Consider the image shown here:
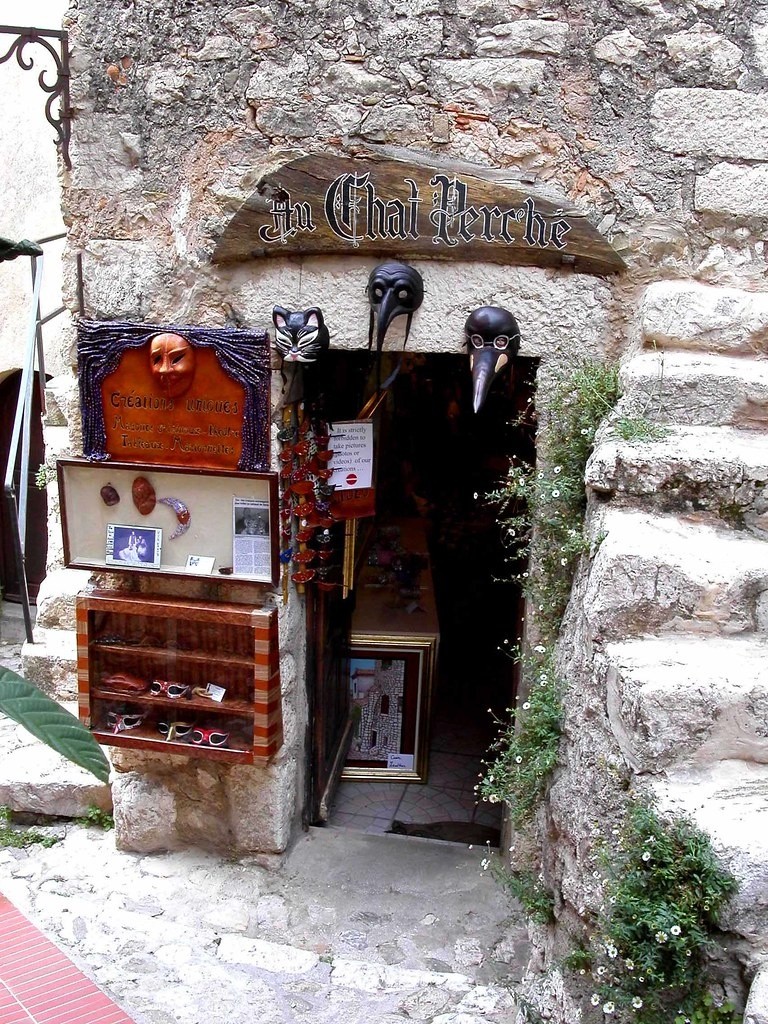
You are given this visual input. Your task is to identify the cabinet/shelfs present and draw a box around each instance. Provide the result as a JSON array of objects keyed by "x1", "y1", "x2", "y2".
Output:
[{"x1": 74, "y1": 595, "x2": 279, "y2": 766}]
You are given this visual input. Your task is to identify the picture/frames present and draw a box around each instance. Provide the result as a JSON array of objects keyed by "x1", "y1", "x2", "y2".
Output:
[{"x1": 341, "y1": 631, "x2": 437, "y2": 785}]
